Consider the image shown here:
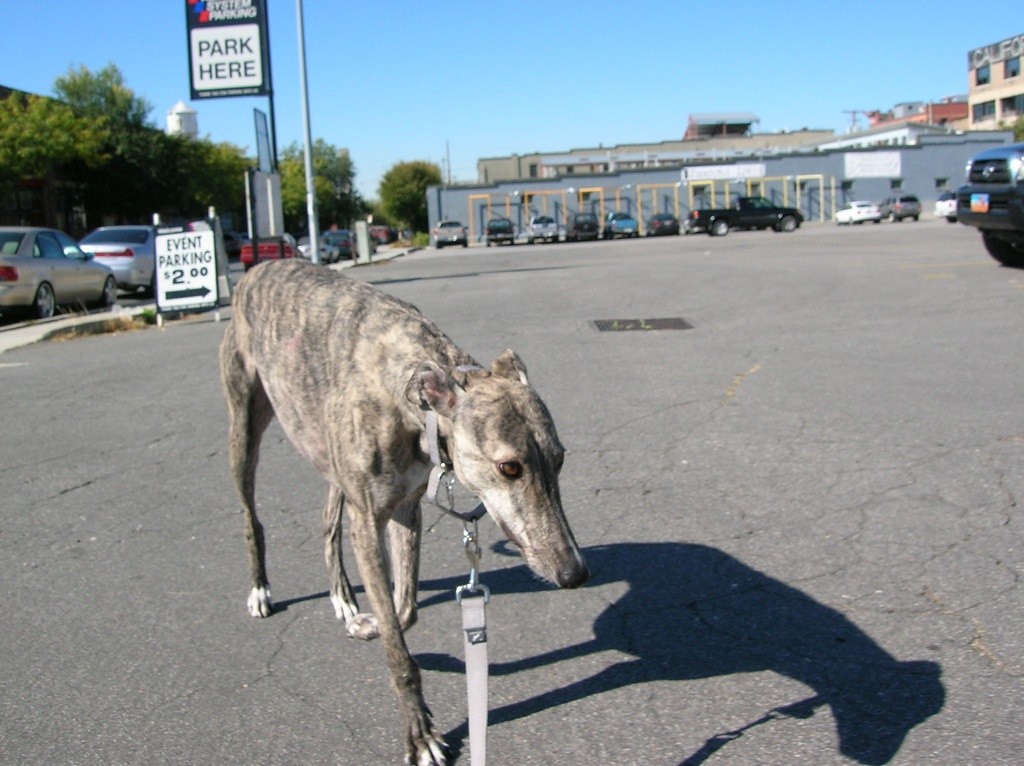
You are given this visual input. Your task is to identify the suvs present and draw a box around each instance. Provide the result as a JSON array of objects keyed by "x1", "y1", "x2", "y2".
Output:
[
  {"x1": 955, "y1": 141, "x2": 1024, "y2": 270},
  {"x1": 878, "y1": 195, "x2": 921, "y2": 223},
  {"x1": 433, "y1": 220, "x2": 468, "y2": 249}
]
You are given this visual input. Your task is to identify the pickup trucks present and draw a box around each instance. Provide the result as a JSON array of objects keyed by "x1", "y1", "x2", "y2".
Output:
[
  {"x1": 935, "y1": 198, "x2": 959, "y2": 223},
  {"x1": 689, "y1": 196, "x2": 804, "y2": 236},
  {"x1": 527, "y1": 216, "x2": 559, "y2": 244},
  {"x1": 602, "y1": 211, "x2": 639, "y2": 240}
]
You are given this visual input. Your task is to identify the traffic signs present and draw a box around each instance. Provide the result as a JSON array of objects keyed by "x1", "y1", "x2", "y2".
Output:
[{"x1": 155, "y1": 231, "x2": 217, "y2": 314}]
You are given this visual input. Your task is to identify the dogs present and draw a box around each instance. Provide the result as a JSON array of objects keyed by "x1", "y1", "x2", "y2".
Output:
[{"x1": 219, "y1": 256, "x2": 593, "y2": 766}]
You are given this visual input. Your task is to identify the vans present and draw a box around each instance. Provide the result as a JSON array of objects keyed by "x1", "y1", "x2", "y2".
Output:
[
  {"x1": 485, "y1": 219, "x2": 515, "y2": 246},
  {"x1": 565, "y1": 211, "x2": 599, "y2": 242}
]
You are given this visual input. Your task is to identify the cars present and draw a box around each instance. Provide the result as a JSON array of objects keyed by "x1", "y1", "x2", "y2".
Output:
[
  {"x1": 835, "y1": 200, "x2": 881, "y2": 226},
  {"x1": 644, "y1": 214, "x2": 679, "y2": 237},
  {"x1": 297, "y1": 225, "x2": 393, "y2": 265},
  {"x1": 241, "y1": 233, "x2": 302, "y2": 272},
  {"x1": 0, "y1": 225, "x2": 118, "y2": 322},
  {"x1": 62, "y1": 225, "x2": 166, "y2": 296}
]
